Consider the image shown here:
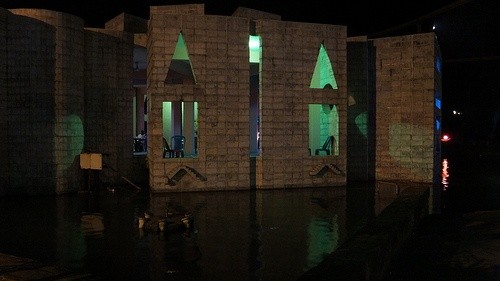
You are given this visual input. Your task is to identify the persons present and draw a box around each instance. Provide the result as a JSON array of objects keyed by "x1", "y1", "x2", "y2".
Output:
[{"x1": 137, "y1": 129, "x2": 146, "y2": 152}]
